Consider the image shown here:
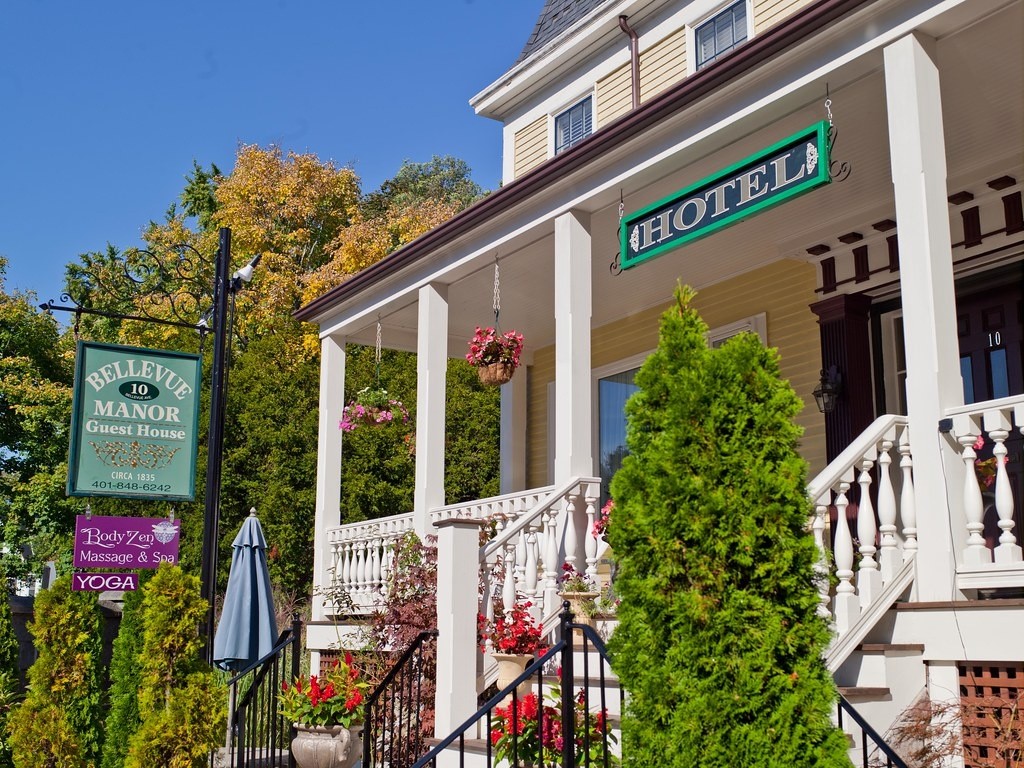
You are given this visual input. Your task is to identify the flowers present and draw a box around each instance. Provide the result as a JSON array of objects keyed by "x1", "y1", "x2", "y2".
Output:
[
  {"x1": 276, "y1": 650, "x2": 373, "y2": 729},
  {"x1": 465, "y1": 327, "x2": 525, "y2": 368},
  {"x1": 592, "y1": 499, "x2": 614, "y2": 540},
  {"x1": 478, "y1": 601, "x2": 549, "y2": 658},
  {"x1": 974, "y1": 436, "x2": 1009, "y2": 516},
  {"x1": 491, "y1": 666, "x2": 619, "y2": 768},
  {"x1": 339, "y1": 386, "x2": 409, "y2": 433},
  {"x1": 556, "y1": 563, "x2": 598, "y2": 618}
]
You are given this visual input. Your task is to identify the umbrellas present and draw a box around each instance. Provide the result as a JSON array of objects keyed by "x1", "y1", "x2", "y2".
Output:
[{"x1": 213, "y1": 506, "x2": 282, "y2": 680}]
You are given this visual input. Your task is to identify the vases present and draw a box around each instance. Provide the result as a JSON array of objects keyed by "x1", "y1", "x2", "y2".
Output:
[
  {"x1": 491, "y1": 653, "x2": 534, "y2": 708},
  {"x1": 559, "y1": 592, "x2": 600, "y2": 643},
  {"x1": 291, "y1": 723, "x2": 364, "y2": 768},
  {"x1": 602, "y1": 536, "x2": 619, "y2": 583},
  {"x1": 362, "y1": 416, "x2": 386, "y2": 427},
  {"x1": 985, "y1": 537, "x2": 994, "y2": 563},
  {"x1": 478, "y1": 363, "x2": 515, "y2": 384}
]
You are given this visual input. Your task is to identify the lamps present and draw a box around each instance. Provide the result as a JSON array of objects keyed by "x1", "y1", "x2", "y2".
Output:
[{"x1": 812, "y1": 365, "x2": 847, "y2": 412}]
